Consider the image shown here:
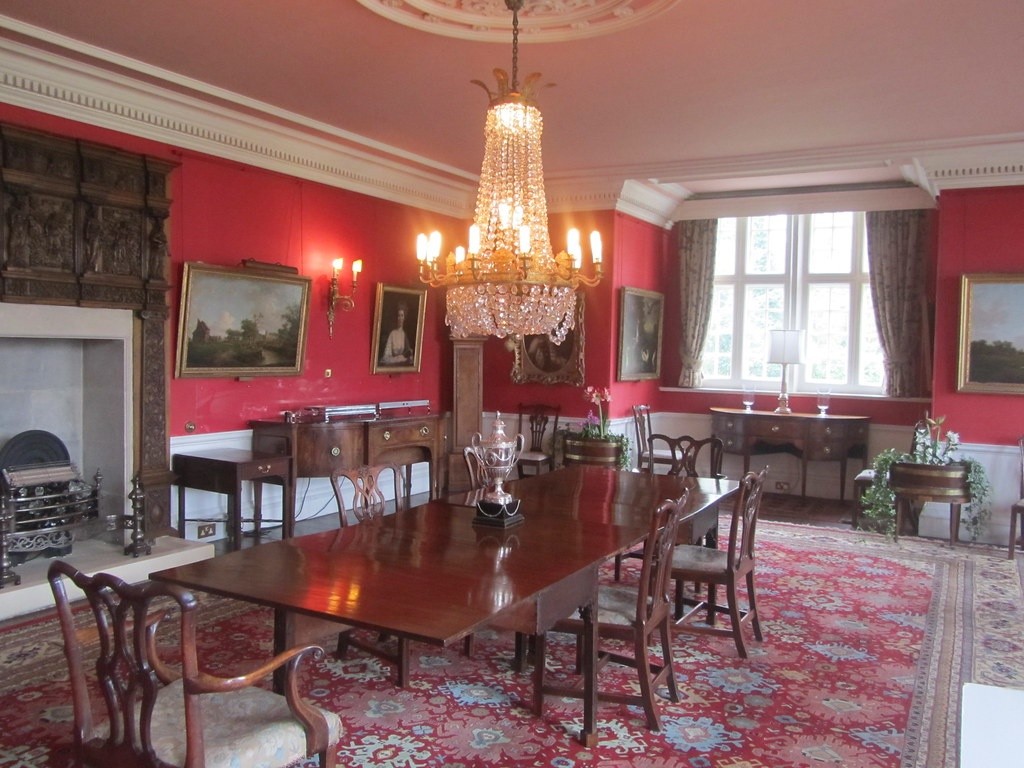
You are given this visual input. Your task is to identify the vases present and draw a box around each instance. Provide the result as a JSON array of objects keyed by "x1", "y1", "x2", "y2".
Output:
[{"x1": 562, "y1": 437, "x2": 622, "y2": 468}]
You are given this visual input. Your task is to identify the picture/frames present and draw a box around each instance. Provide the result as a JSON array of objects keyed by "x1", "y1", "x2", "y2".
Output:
[
  {"x1": 369, "y1": 282, "x2": 427, "y2": 373},
  {"x1": 510, "y1": 292, "x2": 585, "y2": 388},
  {"x1": 174, "y1": 262, "x2": 313, "y2": 377},
  {"x1": 955, "y1": 272, "x2": 1024, "y2": 396},
  {"x1": 617, "y1": 286, "x2": 665, "y2": 381}
]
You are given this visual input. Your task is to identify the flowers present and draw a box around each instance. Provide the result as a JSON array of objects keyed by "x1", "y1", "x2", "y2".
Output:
[{"x1": 549, "y1": 386, "x2": 631, "y2": 458}]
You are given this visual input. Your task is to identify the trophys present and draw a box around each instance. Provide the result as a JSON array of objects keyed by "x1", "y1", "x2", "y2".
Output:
[{"x1": 472, "y1": 411, "x2": 525, "y2": 527}]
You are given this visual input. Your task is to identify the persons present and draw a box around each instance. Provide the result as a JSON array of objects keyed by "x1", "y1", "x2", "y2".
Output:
[
  {"x1": 86, "y1": 212, "x2": 101, "y2": 263},
  {"x1": 10, "y1": 202, "x2": 67, "y2": 265},
  {"x1": 150, "y1": 223, "x2": 171, "y2": 280}
]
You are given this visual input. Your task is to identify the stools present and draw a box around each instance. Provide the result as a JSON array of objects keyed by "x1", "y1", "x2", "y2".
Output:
[
  {"x1": 1008, "y1": 499, "x2": 1024, "y2": 560},
  {"x1": 854, "y1": 468, "x2": 890, "y2": 528}
]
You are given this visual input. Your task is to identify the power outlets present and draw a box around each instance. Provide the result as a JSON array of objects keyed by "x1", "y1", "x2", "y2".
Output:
[
  {"x1": 198, "y1": 524, "x2": 216, "y2": 539},
  {"x1": 775, "y1": 482, "x2": 790, "y2": 490}
]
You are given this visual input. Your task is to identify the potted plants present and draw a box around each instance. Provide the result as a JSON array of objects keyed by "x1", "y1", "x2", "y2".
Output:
[{"x1": 859, "y1": 416, "x2": 993, "y2": 549}]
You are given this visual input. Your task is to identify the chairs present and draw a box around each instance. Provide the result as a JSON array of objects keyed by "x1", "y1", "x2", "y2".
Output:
[
  {"x1": 46, "y1": 563, "x2": 347, "y2": 768},
  {"x1": 331, "y1": 404, "x2": 770, "y2": 731}
]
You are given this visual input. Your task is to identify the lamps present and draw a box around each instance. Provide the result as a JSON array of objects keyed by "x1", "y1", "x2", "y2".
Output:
[
  {"x1": 415, "y1": 0, "x2": 605, "y2": 346},
  {"x1": 327, "y1": 257, "x2": 362, "y2": 339},
  {"x1": 766, "y1": 329, "x2": 801, "y2": 414}
]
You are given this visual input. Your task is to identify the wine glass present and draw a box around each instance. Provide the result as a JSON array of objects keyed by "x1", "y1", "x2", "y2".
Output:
[
  {"x1": 817, "y1": 390, "x2": 830, "y2": 417},
  {"x1": 742, "y1": 384, "x2": 756, "y2": 412}
]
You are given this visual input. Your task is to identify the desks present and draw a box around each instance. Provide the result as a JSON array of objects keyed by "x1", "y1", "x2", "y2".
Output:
[
  {"x1": 147, "y1": 463, "x2": 750, "y2": 748},
  {"x1": 248, "y1": 411, "x2": 448, "y2": 536},
  {"x1": 708, "y1": 407, "x2": 871, "y2": 505},
  {"x1": 172, "y1": 449, "x2": 295, "y2": 553}
]
